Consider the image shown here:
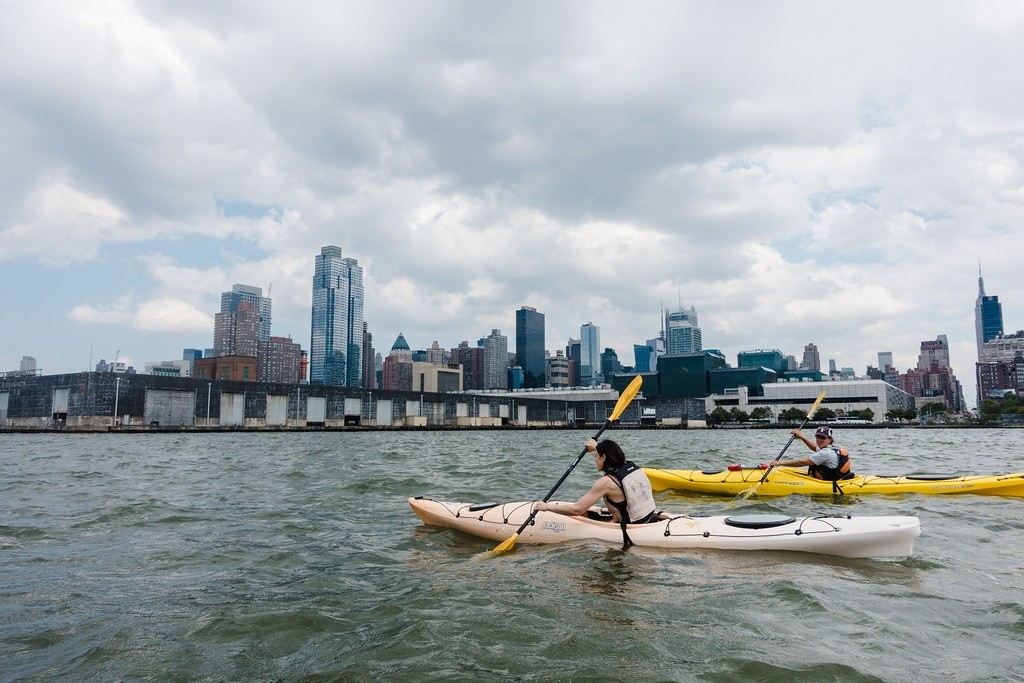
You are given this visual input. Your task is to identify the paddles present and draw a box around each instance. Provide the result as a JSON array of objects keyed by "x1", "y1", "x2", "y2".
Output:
[
  {"x1": 743, "y1": 391, "x2": 826, "y2": 499},
  {"x1": 492, "y1": 373, "x2": 643, "y2": 552}
]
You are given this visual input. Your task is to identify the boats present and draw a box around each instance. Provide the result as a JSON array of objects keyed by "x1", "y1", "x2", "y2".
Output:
[
  {"x1": 642, "y1": 466, "x2": 1024, "y2": 500},
  {"x1": 406, "y1": 496, "x2": 922, "y2": 560}
]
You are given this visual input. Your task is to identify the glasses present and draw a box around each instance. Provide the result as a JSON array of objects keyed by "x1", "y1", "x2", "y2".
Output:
[{"x1": 816, "y1": 435, "x2": 829, "y2": 440}]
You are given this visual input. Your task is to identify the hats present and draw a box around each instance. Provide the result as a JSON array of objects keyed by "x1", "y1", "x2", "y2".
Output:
[{"x1": 814, "y1": 426, "x2": 833, "y2": 439}]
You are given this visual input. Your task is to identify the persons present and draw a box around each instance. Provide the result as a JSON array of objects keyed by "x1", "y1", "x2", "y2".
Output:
[
  {"x1": 770, "y1": 427, "x2": 851, "y2": 481},
  {"x1": 533, "y1": 439, "x2": 658, "y2": 524}
]
